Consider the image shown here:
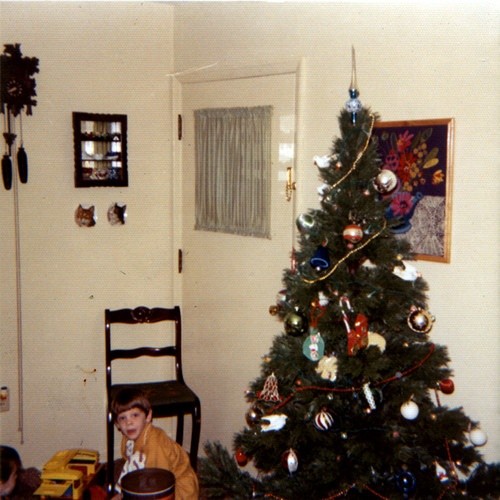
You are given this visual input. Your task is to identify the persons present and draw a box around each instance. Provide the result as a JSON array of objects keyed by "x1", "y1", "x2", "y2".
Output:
[
  {"x1": 0, "y1": 446, "x2": 20, "y2": 500},
  {"x1": 107, "y1": 389, "x2": 199, "y2": 500}
]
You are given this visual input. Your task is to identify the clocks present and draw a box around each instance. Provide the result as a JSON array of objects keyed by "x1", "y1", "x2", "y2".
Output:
[{"x1": 0, "y1": 43, "x2": 39, "y2": 190}]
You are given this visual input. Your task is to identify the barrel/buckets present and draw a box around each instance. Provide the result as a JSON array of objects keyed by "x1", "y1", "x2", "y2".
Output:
[{"x1": 120, "y1": 468, "x2": 176, "y2": 500}]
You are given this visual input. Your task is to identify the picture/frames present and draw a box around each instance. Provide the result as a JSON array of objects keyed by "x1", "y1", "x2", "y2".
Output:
[{"x1": 367, "y1": 118, "x2": 456, "y2": 264}]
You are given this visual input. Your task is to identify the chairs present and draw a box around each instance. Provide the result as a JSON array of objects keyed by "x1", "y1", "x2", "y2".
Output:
[{"x1": 104, "y1": 305, "x2": 202, "y2": 479}]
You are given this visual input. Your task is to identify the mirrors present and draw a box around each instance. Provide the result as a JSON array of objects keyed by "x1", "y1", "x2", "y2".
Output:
[{"x1": 71, "y1": 111, "x2": 129, "y2": 188}]
